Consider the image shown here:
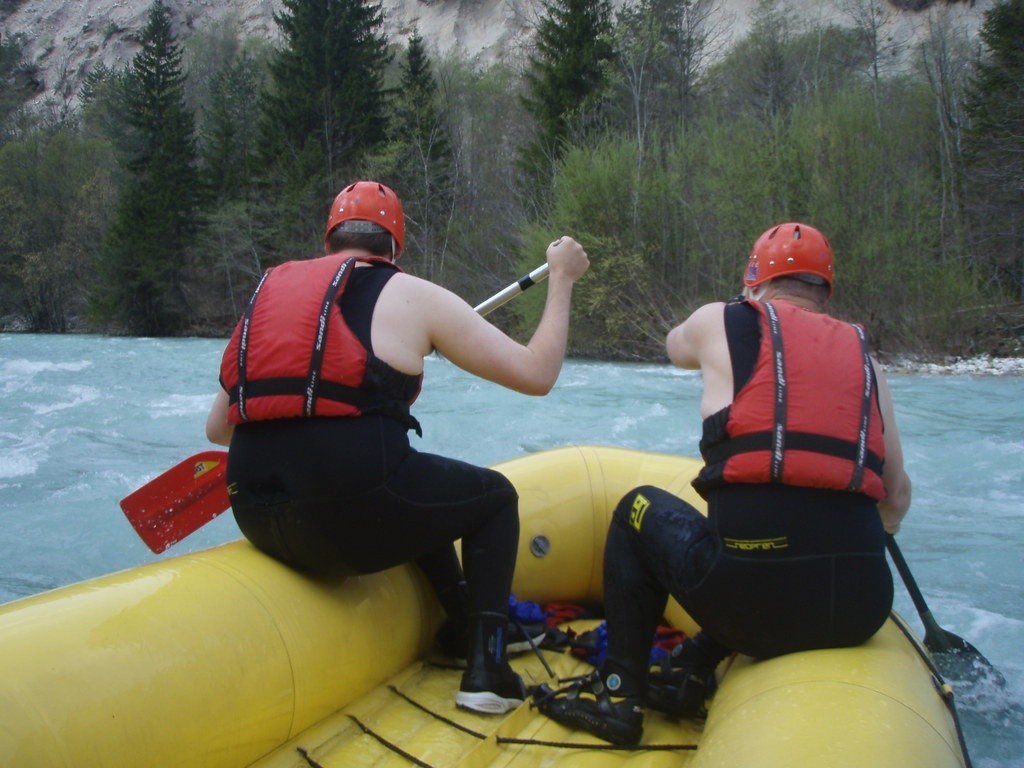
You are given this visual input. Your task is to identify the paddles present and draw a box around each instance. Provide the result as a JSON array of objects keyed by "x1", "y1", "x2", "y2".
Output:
[
  {"x1": 118, "y1": 260, "x2": 549, "y2": 554},
  {"x1": 884, "y1": 531, "x2": 1006, "y2": 691}
]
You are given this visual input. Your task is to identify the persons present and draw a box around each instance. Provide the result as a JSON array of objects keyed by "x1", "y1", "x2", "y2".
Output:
[
  {"x1": 206, "y1": 181, "x2": 589, "y2": 713},
  {"x1": 537, "y1": 223, "x2": 913, "y2": 751}
]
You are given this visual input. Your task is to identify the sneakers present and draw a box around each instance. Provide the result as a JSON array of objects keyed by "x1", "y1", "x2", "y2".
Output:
[
  {"x1": 447, "y1": 620, "x2": 546, "y2": 666},
  {"x1": 534, "y1": 683, "x2": 643, "y2": 746},
  {"x1": 641, "y1": 655, "x2": 706, "y2": 718},
  {"x1": 456, "y1": 665, "x2": 528, "y2": 713}
]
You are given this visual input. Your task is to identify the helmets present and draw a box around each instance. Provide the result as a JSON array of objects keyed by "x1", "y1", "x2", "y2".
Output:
[
  {"x1": 325, "y1": 181, "x2": 404, "y2": 251},
  {"x1": 744, "y1": 222, "x2": 834, "y2": 305}
]
